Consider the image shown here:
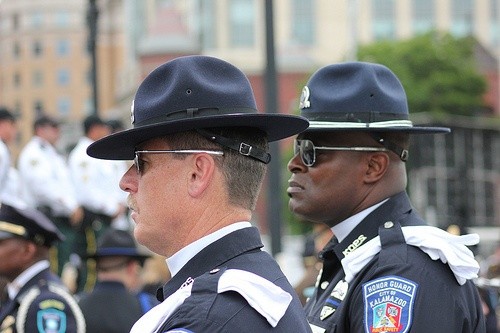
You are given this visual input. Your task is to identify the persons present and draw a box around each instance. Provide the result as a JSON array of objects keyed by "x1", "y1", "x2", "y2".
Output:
[
  {"x1": 40, "y1": 189, "x2": 176, "y2": 333},
  {"x1": 0, "y1": 200, "x2": 87, "y2": 333},
  {"x1": 291, "y1": 209, "x2": 500, "y2": 333},
  {"x1": 105, "y1": 118, "x2": 134, "y2": 219},
  {"x1": 67, "y1": 112, "x2": 125, "y2": 293},
  {"x1": 86, "y1": 54, "x2": 314, "y2": 333},
  {"x1": 284, "y1": 61, "x2": 487, "y2": 333},
  {"x1": 18, "y1": 115, "x2": 84, "y2": 285},
  {"x1": 0, "y1": 106, "x2": 28, "y2": 209}
]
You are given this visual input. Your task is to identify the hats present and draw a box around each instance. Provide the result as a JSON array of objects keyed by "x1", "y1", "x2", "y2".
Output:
[
  {"x1": 0, "y1": 201, "x2": 64, "y2": 246},
  {"x1": 81, "y1": 228, "x2": 155, "y2": 260},
  {"x1": 33, "y1": 116, "x2": 66, "y2": 125},
  {"x1": 301, "y1": 61, "x2": 451, "y2": 132},
  {"x1": 85, "y1": 55, "x2": 309, "y2": 161},
  {"x1": 83, "y1": 114, "x2": 112, "y2": 128},
  {"x1": 0, "y1": 108, "x2": 20, "y2": 121}
]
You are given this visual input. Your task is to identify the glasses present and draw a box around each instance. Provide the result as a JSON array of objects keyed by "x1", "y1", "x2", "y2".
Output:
[
  {"x1": 133, "y1": 145, "x2": 225, "y2": 171},
  {"x1": 293, "y1": 136, "x2": 390, "y2": 165}
]
